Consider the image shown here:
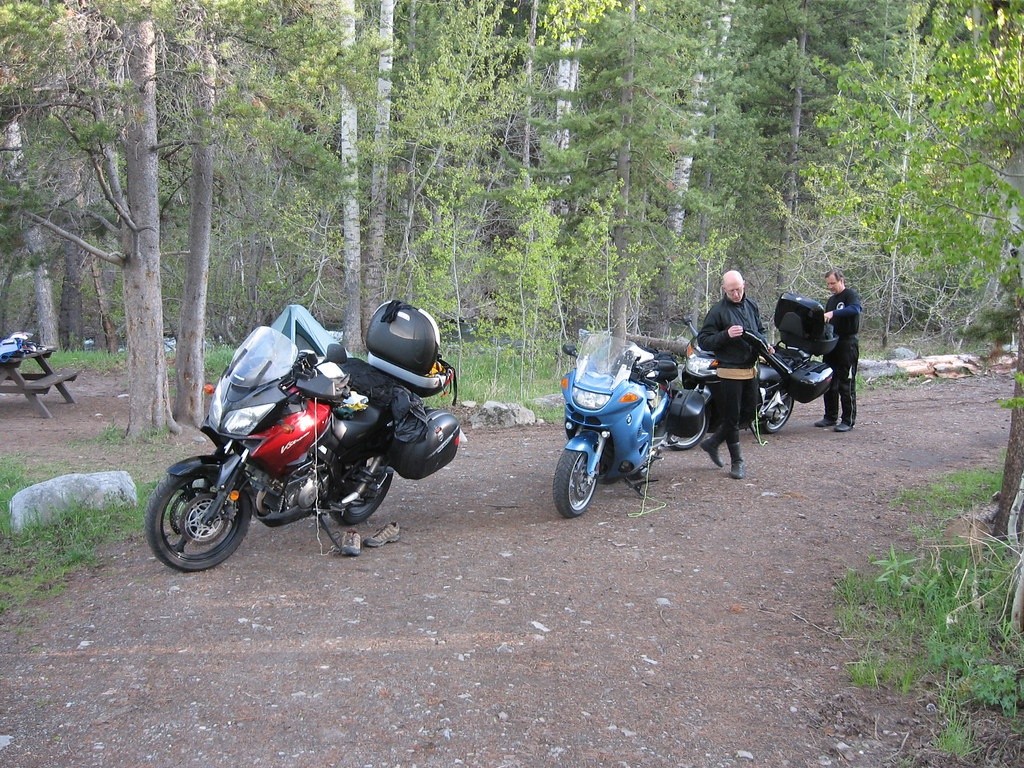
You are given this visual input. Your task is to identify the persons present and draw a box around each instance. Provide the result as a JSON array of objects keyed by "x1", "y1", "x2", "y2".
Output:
[
  {"x1": 696, "y1": 270, "x2": 775, "y2": 479},
  {"x1": 814, "y1": 268, "x2": 862, "y2": 432}
]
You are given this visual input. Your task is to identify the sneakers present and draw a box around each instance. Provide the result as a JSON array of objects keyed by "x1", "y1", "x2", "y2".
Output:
[
  {"x1": 814, "y1": 418, "x2": 838, "y2": 427},
  {"x1": 834, "y1": 422, "x2": 853, "y2": 432},
  {"x1": 363, "y1": 521, "x2": 401, "y2": 547},
  {"x1": 338, "y1": 528, "x2": 361, "y2": 556}
]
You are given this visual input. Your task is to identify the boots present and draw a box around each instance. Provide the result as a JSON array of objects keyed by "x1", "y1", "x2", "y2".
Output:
[
  {"x1": 725, "y1": 441, "x2": 745, "y2": 479},
  {"x1": 699, "y1": 425, "x2": 727, "y2": 468}
]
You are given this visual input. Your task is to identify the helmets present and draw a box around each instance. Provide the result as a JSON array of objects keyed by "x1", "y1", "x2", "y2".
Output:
[{"x1": 9, "y1": 332, "x2": 34, "y2": 354}]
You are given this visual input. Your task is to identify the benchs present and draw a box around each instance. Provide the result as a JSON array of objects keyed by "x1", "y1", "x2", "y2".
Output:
[{"x1": 23, "y1": 368, "x2": 82, "y2": 394}]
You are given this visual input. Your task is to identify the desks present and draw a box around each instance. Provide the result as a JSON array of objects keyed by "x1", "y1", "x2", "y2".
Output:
[{"x1": 0, "y1": 346, "x2": 77, "y2": 418}]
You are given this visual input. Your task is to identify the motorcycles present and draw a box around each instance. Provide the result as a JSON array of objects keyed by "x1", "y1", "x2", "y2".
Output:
[
  {"x1": 670, "y1": 296, "x2": 841, "y2": 436},
  {"x1": 0, "y1": 329, "x2": 46, "y2": 354},
  {"x1": 144, "y1": 296, "x2": 463, "y2": 574},
  {"x1": 550, "y1": 327, "x2": 708, "y2": 520}
]
